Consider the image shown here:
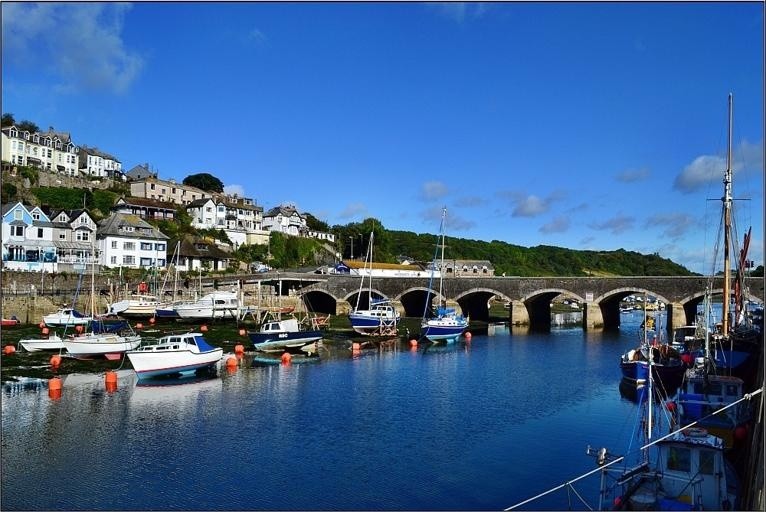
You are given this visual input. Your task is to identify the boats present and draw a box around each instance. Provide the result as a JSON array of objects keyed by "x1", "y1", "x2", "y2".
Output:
[
  {"x1": 125, "y1": 333, "x2": 224, "y2": 381},
  {"x1": 420, "y1": 206, "x2": 470, "y2": 345},
  {"x1": 19, "y1": 240, "x2": 142, "y2": 361},
  {"x1": 111, "y1": 239, "x2": 296, "y2": 321},
  {"x1": 348, "y1": 232, "x2": 401, "y2": 336},
  {"x1": 246, "y1": 283, "x2": 332, "y2": 354}
]
